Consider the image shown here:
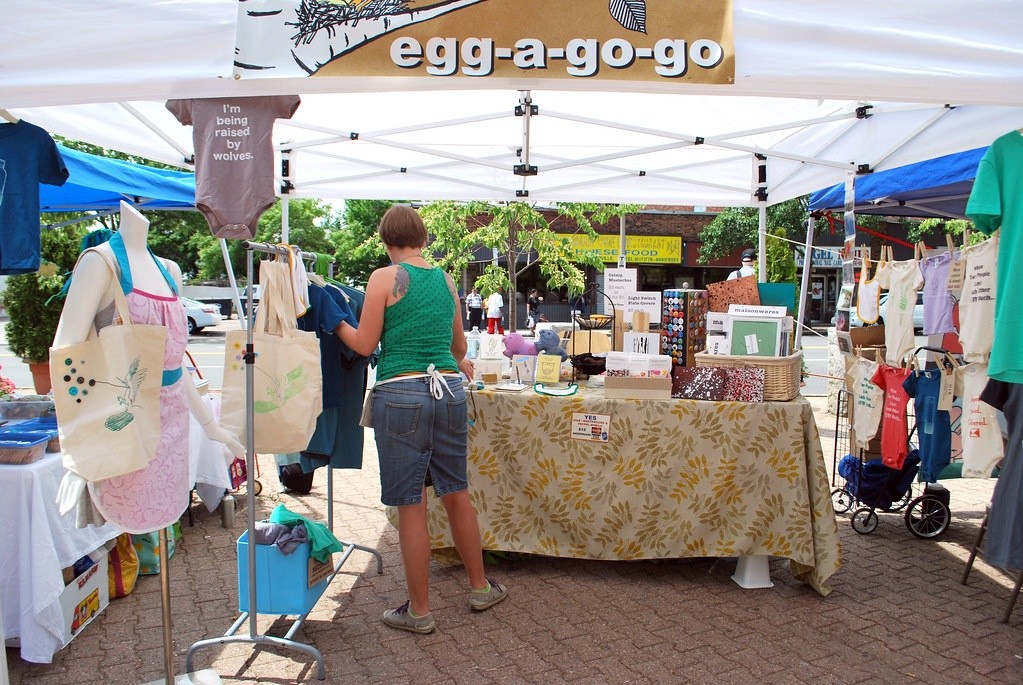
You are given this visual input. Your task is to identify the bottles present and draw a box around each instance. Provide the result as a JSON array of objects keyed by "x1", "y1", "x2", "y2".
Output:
[
  {"x1": 469, "y1": 327, "x2": 480, "y2": 339},
  {"x1": 221, "y1": 488, "x2": 235, "y2": 528}
]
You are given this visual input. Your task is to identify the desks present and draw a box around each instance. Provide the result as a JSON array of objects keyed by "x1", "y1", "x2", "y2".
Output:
[
  {"x1": 0, "y1": 392, "x2": 235, "y2": 663},
  {"x1": 385, "y1": 375, "x2": 841, "y2": 598}
]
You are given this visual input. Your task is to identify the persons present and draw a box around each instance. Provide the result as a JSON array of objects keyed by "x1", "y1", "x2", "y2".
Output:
[
  {"x1": 565, "y1": 290, "x2": 585, "y2": 314},
  {"x1": 465, "y1": 286, "x2": 482, "y2": 333},
  {"x1": 526, "y1": 289, "x2": 543, "y2": 335},
  {"x1": 481, "y1": 292, "x2": 504, "y2": 335},
  {"x1": 54, "y1": 199, "x2": 248, "y2": 530},
  {"x1": 726, "y1": 249, "x2": 757, "y2": 279},
  {"x1": 334, "y1": 205, "x2": 507, "y2": 634}
]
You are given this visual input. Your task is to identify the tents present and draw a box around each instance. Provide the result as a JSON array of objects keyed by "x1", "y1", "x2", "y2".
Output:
[
  {"x1": 37, "y1": 145, "x2": 248, "y2": 330},
  {"x1": 0, "y1": 0, "x2": 1023, "y2": 347},
  {"x1": 794, "y1": 146, "x2": 989, "y2": 350}
]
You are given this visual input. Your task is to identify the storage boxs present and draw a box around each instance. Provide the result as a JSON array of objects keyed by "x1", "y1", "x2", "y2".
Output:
[
  {"x1": 604, "y1": 375, "x2": 672, "y2": 400},
  {"x1": 59, "y1": 537, "x2": 118, "y2": 650},
  {"x1": 844, "y1": 325, "x2": 909, "y2": 463},
  {"x1": 237, "y1": 519, "x2": 327, "y2": 615}
]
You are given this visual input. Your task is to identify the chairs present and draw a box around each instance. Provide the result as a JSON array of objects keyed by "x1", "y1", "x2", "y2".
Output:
[{"x1": 960, "y1": 432, "x2": 1023, "y2": 624}]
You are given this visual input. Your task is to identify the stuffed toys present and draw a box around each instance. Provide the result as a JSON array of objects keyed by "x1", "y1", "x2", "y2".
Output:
[{"x1": 502, "y1": 329, "x2": 567, "y2": 362}]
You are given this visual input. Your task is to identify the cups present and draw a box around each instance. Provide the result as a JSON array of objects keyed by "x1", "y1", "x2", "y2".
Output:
[{"x1": 466, "y1": 339, "x2": 477, "y2": 359}]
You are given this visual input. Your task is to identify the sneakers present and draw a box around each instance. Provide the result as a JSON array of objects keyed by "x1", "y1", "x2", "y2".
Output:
[
  {"x1": 468, "y1": 577, "x2": 509, "y2": 611},
  {"x1": 381, "y1": 600, "x2": 436, "y2": 634}
]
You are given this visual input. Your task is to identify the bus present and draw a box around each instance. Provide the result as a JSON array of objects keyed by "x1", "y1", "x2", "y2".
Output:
[{"x1": 182, "y1": 296, "x2": 223, "y2": 335}]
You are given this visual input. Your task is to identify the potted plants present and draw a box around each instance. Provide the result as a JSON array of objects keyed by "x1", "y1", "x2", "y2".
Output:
[{"x1": 0, "y1": 216, "x2": 90, "y2": 397}]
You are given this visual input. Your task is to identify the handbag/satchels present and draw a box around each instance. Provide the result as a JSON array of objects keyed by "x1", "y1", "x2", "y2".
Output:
[
  {"x1": 49, "y1": 247, "x2": 167, "y2": 481},
  {"x1": 219, "y1": 263, "x2": 323, "y2": 453},
  {"x1": 525, "y1": 316, "x2": 535, "y2": 328},
  {"x1": 360, "y1": 384, "x2": 379, "y2": 428}
]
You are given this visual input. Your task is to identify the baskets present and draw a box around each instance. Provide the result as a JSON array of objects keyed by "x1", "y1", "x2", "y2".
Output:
[
  {"x1": 696, "y1": 350, "x2": 803, "y2": 401},
  {"x1": 0, "y1": 428, "x2": 61, "y2": 465}
]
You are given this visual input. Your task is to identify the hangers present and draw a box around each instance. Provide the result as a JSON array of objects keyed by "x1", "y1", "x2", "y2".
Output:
[{"x1": 259, "y1": 242, "x2": 346, "y2": 288}]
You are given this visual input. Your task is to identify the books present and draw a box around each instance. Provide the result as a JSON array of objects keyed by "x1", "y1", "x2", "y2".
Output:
[{"x1": 706, "y1": 312, "x2": 790, "y2": 357}]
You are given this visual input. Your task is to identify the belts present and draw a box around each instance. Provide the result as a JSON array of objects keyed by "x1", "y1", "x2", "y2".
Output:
[{"x1": 393, "y1": 369, "x2": 454, "y2": 380}]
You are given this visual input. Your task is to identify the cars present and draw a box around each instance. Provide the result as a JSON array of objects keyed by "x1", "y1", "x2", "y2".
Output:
[
  {"x1": 241, "y1": 284, "x2": 261, "y2": 314},
  {"x1": 831, "y1": 291, "x2": 925, "y2": 328}
]
